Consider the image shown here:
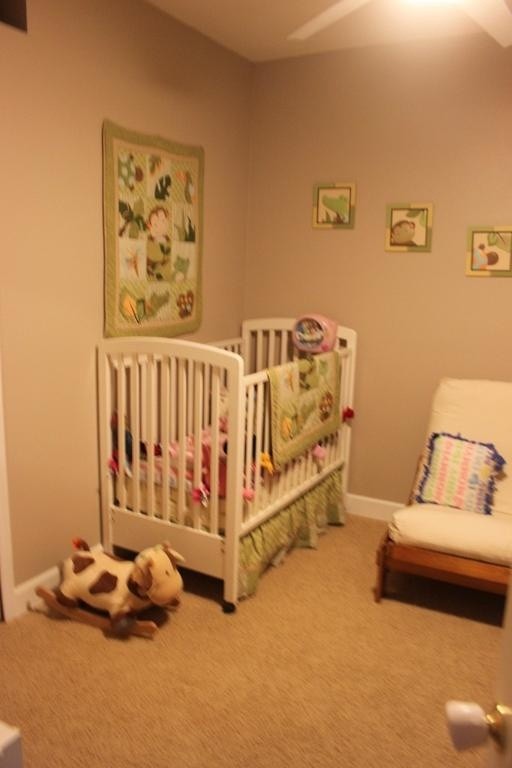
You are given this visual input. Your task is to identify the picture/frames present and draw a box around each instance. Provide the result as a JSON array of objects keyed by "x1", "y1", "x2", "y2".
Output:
[
  {"x1": 465, "y1": 224, "x2": 512, "y2": 278},
  {"x1": 312, "y1": 182, "x2": 356, "y2": 230},
  {"x1": 384, "y1": 203, "x2": 434, "y2": 252}
]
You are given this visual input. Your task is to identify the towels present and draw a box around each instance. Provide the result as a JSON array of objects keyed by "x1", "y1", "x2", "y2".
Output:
[{"x1": 267, "y1": 350, "x2": 344, "y2": 472}]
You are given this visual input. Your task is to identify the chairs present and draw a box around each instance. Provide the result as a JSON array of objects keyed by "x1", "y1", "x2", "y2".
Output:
[{"x1": 370, "y1": 378, "x2": 512, "y2": 627}]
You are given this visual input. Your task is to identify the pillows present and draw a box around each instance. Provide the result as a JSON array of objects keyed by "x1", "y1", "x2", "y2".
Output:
[{"x1": 416, "y1": 431, "x2": 506, "y2": 515}]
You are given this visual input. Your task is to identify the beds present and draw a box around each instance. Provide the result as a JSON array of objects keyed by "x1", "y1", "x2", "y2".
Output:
[{"x1": 96, "y1": 316, "x2": 357, "y2": 614}]
